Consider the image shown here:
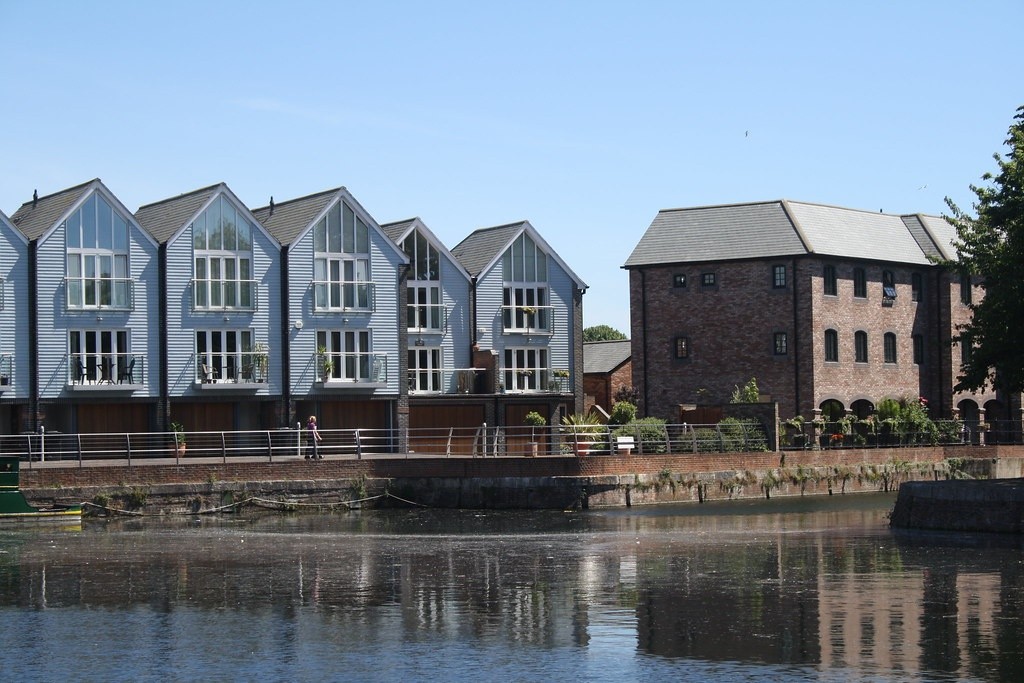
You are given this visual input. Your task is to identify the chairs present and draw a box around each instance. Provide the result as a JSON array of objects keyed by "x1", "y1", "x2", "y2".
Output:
[
  {"x1": 202, "y1": 364, "x2": 219, "y2": 384},
  {"x1": 237, "y1": 363, "x2": 256, "y2": 383},
  {"x1": 76, "y1": 360, "x2": 96, "y2": 385},
  {"x1": 120, "y1": 360, "x2": 135, "y2": 384}
]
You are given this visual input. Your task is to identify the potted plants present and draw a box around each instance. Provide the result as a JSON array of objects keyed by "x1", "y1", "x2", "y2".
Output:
[
  {"x1": 169, "y1": 421, "x2": 186, "y2": 458},
  {"x1": 523, "y1": 411, "x2": 546, "y2": 456},
  {"x1": 612, "y1": 401, "x2": 638, "y2": 455},
  {"x1": 563, "y1": 411, "x2": 602, "y2": 456},
  {"x1": 321, "y1": 360, "x2": 335, "y2": 382},
  {"x1": 252, "y1": 341, "x2": 271, "y2": 383}
]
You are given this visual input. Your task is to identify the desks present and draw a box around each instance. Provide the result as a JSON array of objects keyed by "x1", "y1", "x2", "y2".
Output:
[
  {"x1": 97, "y1": 364, "x2": 116, "y2": 385},
  {"x1": 222, "y1": 366, "x2": 240, "y2": 383}
]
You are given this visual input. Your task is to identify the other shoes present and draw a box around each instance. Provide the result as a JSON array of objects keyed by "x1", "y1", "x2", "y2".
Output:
[
  {"x1": 319, "y1": 455, "x2": 323, "y2": 459},
  {"x1": 305, "y1": 456, "x2": 310, "y2": 459}
]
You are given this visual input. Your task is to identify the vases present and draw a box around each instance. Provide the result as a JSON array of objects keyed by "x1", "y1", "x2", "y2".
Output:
[{"x1": 834, "y1": 442, "x2": 844, "y2": 447}]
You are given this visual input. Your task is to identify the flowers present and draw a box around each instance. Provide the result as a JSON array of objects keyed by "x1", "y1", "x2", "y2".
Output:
[{"x1": 831, "y1": 435, "x2": 844, "y2": 442}]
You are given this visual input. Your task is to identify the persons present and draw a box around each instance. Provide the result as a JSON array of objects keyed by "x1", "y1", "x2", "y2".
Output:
[{"x1": 304, "y1": 416, "x2": 325, "y2": 459}]
